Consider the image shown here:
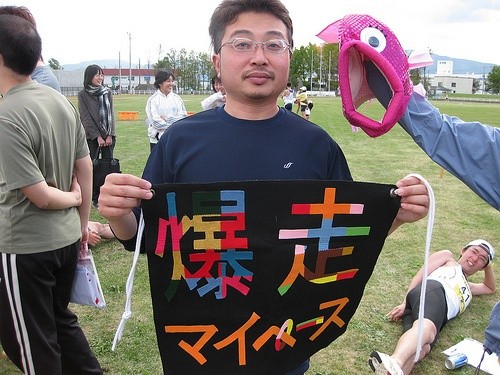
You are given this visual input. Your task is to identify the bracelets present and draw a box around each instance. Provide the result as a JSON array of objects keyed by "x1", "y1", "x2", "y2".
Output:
[{"x1": 484, "y1": 262, "x2": 495, "y2": 272}]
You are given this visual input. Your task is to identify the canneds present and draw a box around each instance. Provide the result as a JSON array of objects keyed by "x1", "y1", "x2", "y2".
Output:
[{"x1": 444, "y1": 353, "x2": 468, "y2": 370}]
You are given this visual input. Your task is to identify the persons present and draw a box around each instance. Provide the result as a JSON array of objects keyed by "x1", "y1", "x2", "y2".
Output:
[
  {"x1": 144, "y1": 71, "x2": 188, "y2": 153},
  {"x1": 98, "y1": 0, "x2": 429, "y2": 375},
  {"x1": 368, "y1": 238, "x2": 497, "y2": 375},
  {"x1": 0, "y1": 5, "x2": 63, "y2": 93},
  {"x1": 77, "y1": 64, "x2": 117, "y2": 208},
  {"x1": 358, "y1": 47, "x2": 500, "y2": 375},
  {"x1": 0, "y1": 14, "x2": 104, "y2": 375},
  {"x1": 211, "y1": 75, "x2": 227, "y2": 109},
  {"x1": 281, "y1": 83, "x2": 313, "y2": 120}
]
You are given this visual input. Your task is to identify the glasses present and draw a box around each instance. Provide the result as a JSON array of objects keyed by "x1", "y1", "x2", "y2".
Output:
[
  {"x1": 217, "y1": 37, "x2": 293, "y2": 54},
  {"x1": 92, "y1": 74, "x2": 104, "y2": 78}
]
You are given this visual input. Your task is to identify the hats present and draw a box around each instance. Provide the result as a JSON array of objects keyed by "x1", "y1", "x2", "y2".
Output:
[{"x1": 458, "y1": 240, "x2": 496, "y2": 272}]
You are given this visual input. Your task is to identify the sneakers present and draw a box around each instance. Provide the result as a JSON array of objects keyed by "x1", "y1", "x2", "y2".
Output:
[{"x1": 367, "y1": 350, "x2": 405, "y2": 375}]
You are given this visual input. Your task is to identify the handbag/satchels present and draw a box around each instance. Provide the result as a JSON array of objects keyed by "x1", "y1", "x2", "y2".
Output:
[{"x1": 91, "y1": 143, "x2": 122, "y2": 188}]
